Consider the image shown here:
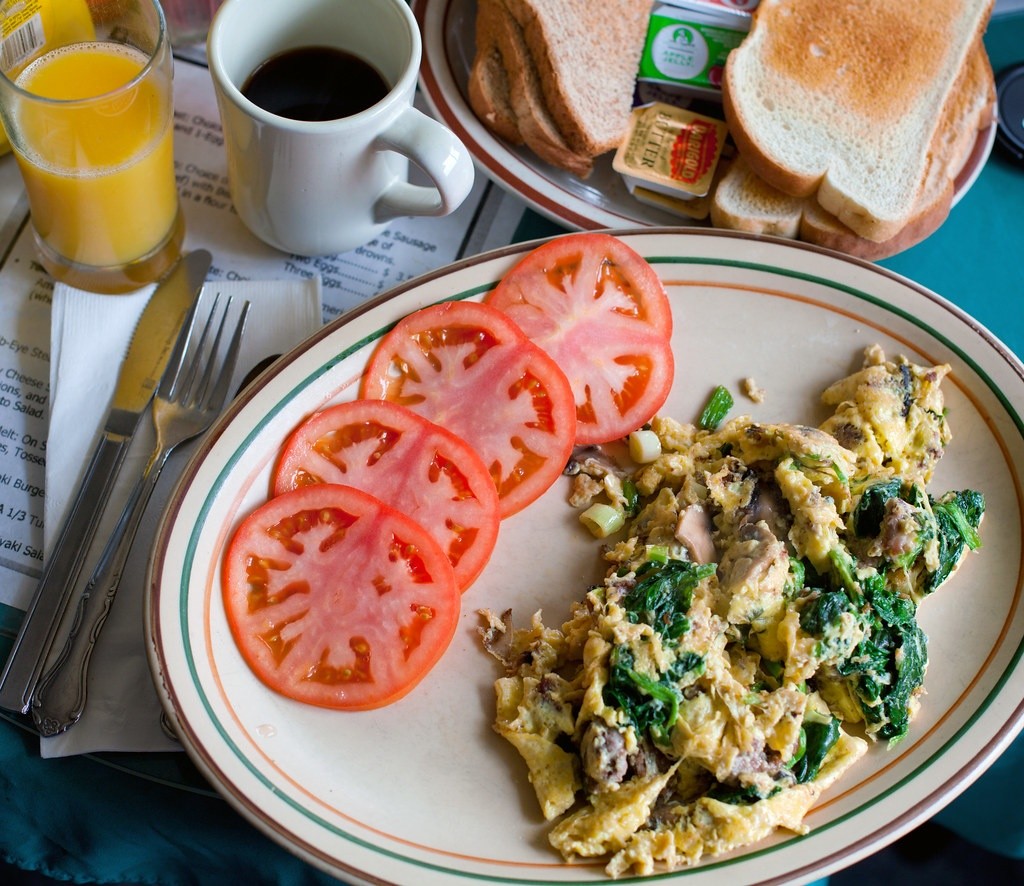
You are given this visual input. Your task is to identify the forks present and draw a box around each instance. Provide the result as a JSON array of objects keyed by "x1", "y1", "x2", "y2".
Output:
[{"x1": 30, "y1": 286, "x2": 251, "y2": 743}]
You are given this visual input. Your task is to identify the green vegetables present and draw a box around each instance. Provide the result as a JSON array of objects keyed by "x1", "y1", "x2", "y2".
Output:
[{"x1": 605, "y1": 476, "x2": 987, "y2": 805}]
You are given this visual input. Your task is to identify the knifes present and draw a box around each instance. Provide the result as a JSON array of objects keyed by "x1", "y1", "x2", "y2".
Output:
[{"x1": 1, "y1": 250, "x2": 211, "y2": 713}]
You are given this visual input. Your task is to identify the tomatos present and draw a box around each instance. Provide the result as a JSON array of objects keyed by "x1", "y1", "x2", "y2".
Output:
[{"x1": 220, "y1": 233, "x2": 675, "y2": 709}]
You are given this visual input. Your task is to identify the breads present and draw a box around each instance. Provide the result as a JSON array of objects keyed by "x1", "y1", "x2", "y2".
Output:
[{"x1": 466, "y1": 0, "x2": 998, "y2": 262}]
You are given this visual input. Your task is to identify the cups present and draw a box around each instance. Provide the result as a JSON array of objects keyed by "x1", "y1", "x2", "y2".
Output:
[
  {"x1": 207, "y1": 0, "x2": 475, "y2": 258},
  {"x1": 1, "y1": 0, "x2": 186, "y2": 295}
]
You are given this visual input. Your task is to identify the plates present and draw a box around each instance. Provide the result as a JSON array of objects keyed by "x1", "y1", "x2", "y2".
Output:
[
  {"x1": 409, "y1": 0, "x2": 1000, "y2": 260},
  {"x1": 146, "y1": 227, "x2": 1023, "y2": 886}
]
